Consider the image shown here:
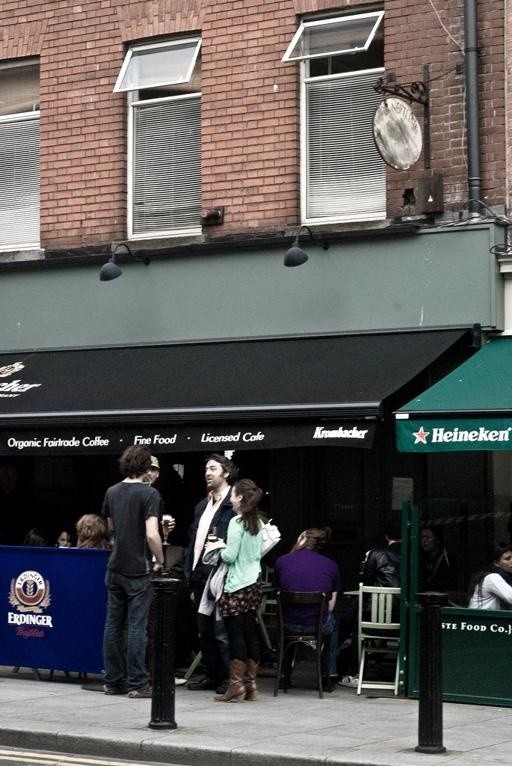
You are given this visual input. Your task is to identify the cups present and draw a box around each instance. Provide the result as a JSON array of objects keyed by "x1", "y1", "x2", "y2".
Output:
[{"x1": 207, "y1": 533, "x2": 218, "y2": 542}]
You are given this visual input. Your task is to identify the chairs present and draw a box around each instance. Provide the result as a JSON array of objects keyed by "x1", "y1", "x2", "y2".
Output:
[{"x1": 168, "y1": 566, "x2": 408, "y2": 699}]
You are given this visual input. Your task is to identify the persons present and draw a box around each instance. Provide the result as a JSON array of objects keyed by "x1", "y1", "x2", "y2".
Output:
[
  {"x1": 419, "y1": 528, "x2": 469, "y2": 593},
  {"x1": 466, "y1": 542, "x2": 512, "y2": 610},
  {"x1": 56, "y1": 446, "x2": 403, "y2": 702},
  {"x1": 24, "y1": 527, "x2": 51, "y2": 547}
]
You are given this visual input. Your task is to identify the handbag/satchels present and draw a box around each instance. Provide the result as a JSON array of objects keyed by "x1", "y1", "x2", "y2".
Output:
[{"x1": 260, "y1": 522, "x2": 282, "y2": 557}]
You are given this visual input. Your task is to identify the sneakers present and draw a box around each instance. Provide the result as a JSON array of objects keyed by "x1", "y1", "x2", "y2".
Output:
[
  {"x1": 104, "y1": 685, "x2": 131, "y2": 694},
  {"x1": 338, "y1": 676, "x2": 358, "y2": 688},
  {"x1": 129, "y1": 687, "x2": 153, "y2": 698},
  {"x1": 322, "y1": 677, "x2": 334, "y2": 691},
  {"x1": 279, "y1": 677, "x2": 291, "y2": 689}
]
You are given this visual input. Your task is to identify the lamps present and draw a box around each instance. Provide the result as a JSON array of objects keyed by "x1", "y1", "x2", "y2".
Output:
[
  {"x1": 281, "y1": 224, "x2": 330, "y2": 266},
  {"x1": 96, "y1": 243, "x2": 152, "y2": 282}
]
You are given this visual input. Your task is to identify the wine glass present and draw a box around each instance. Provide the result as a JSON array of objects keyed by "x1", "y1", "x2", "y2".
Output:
[{"x1": 161, "y1": 516, "x2": 172, "y2": 546}]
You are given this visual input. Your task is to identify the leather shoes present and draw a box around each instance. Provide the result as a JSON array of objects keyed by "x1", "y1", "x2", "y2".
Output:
[
  {"x1": 175, "y1": 676, "x2": 187, "y2": 685},
  {"x1": 188, "y1": 675, "x2": 217, "y2": 689}
]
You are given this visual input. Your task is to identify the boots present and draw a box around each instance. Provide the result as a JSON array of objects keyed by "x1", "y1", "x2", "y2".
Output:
[{"x1": 215, "y1": 658, "x2": 260, "y2": 701}]
